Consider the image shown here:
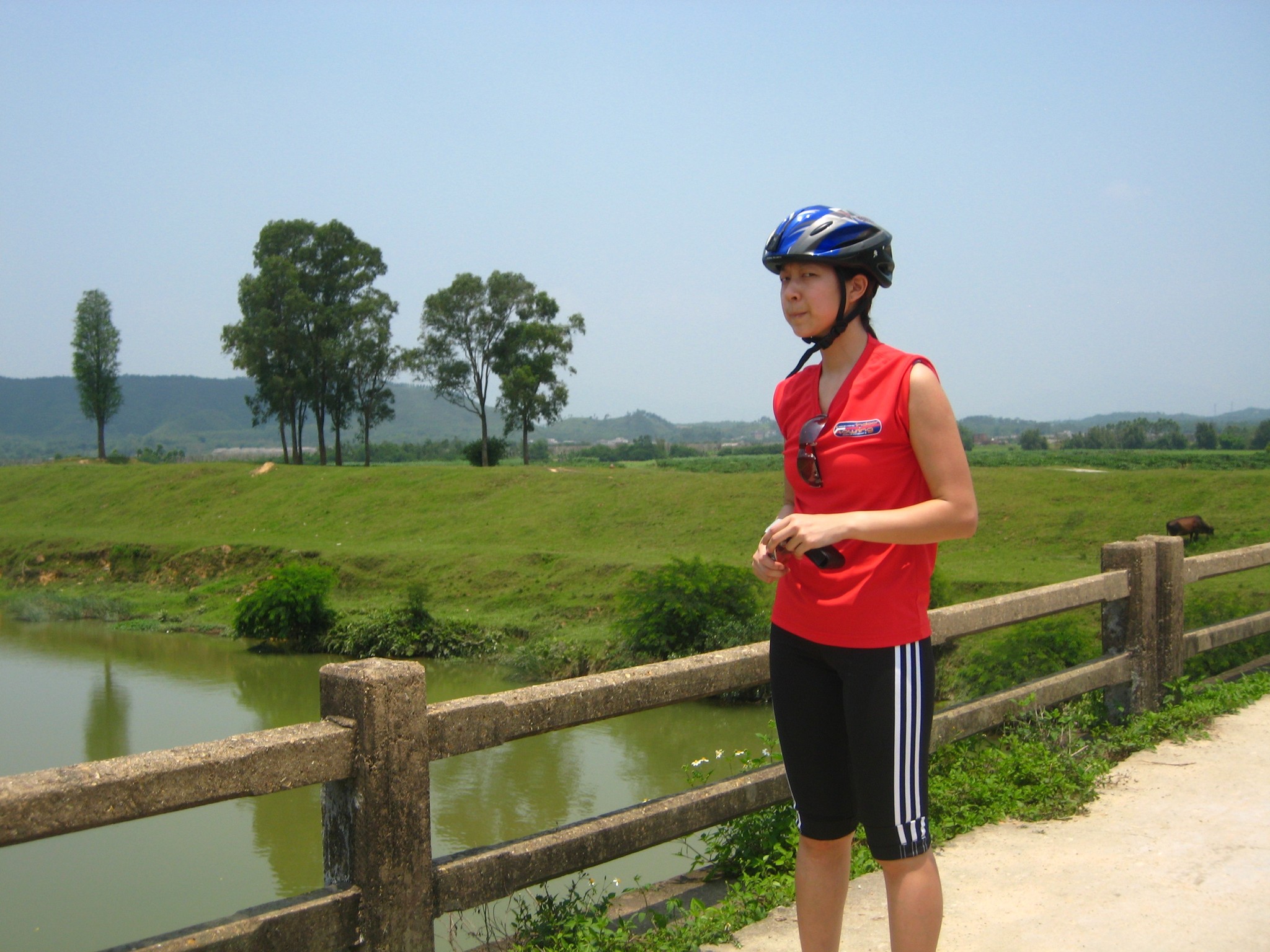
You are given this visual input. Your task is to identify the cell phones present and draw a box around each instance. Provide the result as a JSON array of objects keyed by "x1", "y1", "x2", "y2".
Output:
[{"x1": 786, "y1": 538, "x2": 846, "y2": 570}]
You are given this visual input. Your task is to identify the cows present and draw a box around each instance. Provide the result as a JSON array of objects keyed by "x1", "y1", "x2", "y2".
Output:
[{"x1": 1166, "y1": 515, "x2": 1214, "y2": 543}]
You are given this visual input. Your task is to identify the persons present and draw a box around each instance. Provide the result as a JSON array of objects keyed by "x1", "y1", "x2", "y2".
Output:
[{"x1": 751, "y1": 204, "x2": 979, "y2": 952}]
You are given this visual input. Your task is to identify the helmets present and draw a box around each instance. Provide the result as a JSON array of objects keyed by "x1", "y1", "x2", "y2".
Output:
[{"x1": 762, "y1": 205, "x2": 896, "y2": 288}]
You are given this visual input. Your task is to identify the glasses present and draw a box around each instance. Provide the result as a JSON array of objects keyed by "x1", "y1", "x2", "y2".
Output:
[{"x1": 797, "y1": 414, "x2": 829, "y2": 489}]
